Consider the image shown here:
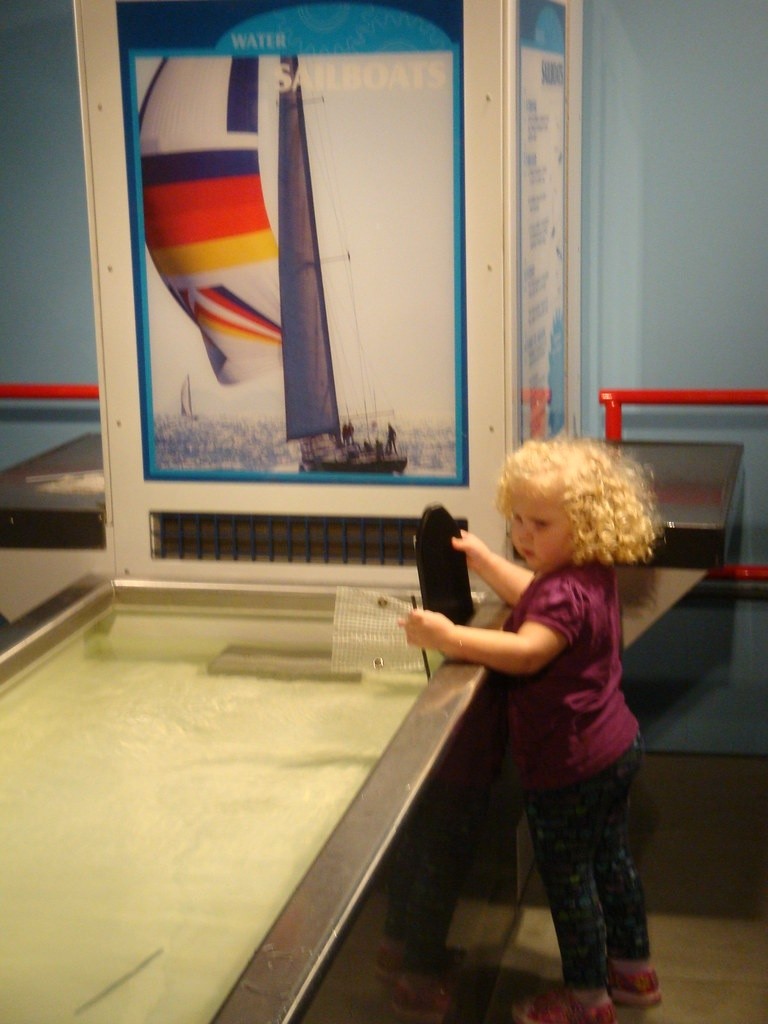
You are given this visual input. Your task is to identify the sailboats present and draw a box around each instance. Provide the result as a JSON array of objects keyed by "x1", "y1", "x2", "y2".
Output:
[{"x1": 138, "y1": 55, "x2": 408, "y2": 472}]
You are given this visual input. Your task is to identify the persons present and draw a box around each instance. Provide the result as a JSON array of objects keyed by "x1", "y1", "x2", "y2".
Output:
[{"x1": 396, "y1": 437, "x2": 664, "y2": 1024}]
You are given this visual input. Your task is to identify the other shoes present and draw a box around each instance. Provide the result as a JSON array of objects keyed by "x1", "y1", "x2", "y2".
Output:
[
  {"x1": 511, "y1": 988, "x2": 618, "y2": 1024},
  {"x1": 607, "y1": 960, "x2": 662, "y2": 1007}
]
